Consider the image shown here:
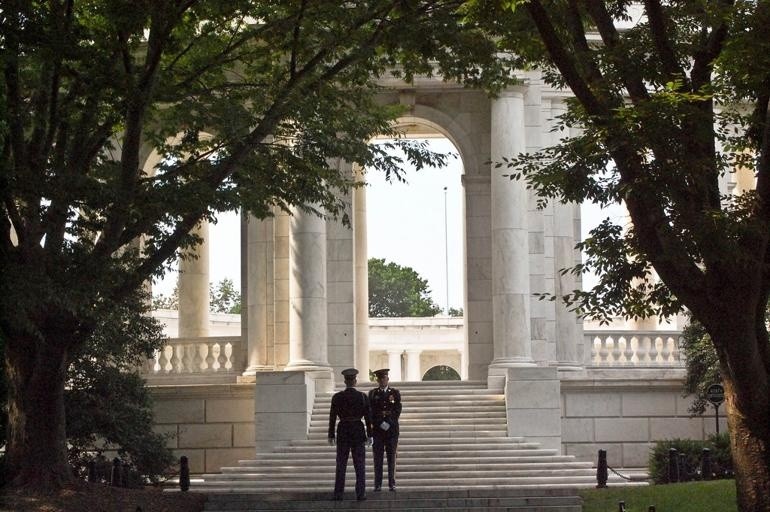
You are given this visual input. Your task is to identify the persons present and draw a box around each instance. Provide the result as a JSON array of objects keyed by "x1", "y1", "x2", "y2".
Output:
[
  {"x1": 328, "y1": 369, "x2": 374, "y2": 501},
  {"x1": 368, "y1": 369, "x2": 402, "y2": 492}
]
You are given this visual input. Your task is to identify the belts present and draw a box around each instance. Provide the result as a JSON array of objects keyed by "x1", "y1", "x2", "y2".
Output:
[
  {"x1": 339, "y1": 417, "x2": 361, "y2": 422},
  {"x1": 375, "y1": 410, "x2": 392, "y2": 415}
]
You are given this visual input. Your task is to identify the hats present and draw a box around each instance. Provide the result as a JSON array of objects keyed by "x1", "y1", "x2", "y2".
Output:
[
  {"x1": 341, "y1": 369, "x2": 359, "y2": 379},
  {"x1": 373, "y1": 369, "x2": 390, "y2": 377}
]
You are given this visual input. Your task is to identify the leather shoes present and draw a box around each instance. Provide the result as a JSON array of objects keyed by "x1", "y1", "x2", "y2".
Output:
[
  {"x1": 357, "y1": 493, "x2": 367, "y2": 500},
  {"x1": 331, "y1": 494, "x2": 342, "y2": 501},
  {"x1": 373, "y1": 484, "x2": 381, "y2": 491},
  {"x1": 389, "y1": 484, "x2": 397, "y2": 490}
]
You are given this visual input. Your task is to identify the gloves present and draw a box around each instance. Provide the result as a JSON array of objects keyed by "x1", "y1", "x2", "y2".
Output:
[
  {"x1": 367, "y1": 436, "x2": 373, "y2": 448},
  {"x1": 328, "y1": 437, "x2": 336, "y2": 446},
  {"x1": 380, "y1": 421, "x2": 390, "y2": 431}
]
